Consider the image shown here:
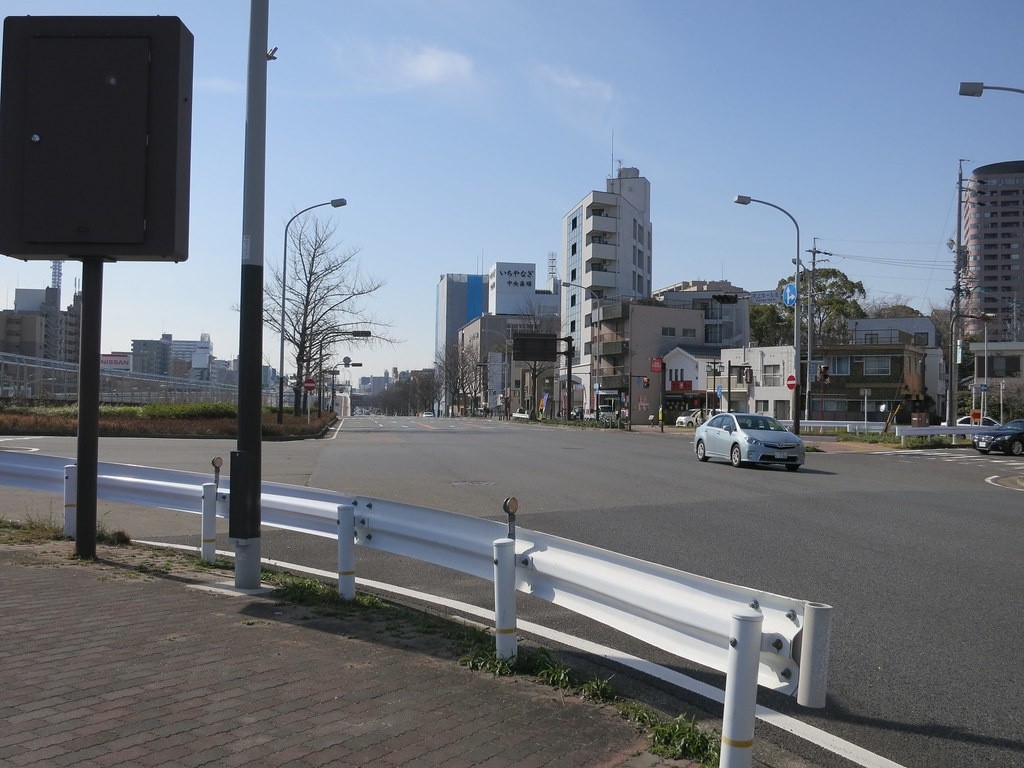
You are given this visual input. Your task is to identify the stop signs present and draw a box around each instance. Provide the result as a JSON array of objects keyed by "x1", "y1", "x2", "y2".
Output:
[
  {"x1": 786, "y1": 375, "x2": 797, "y2": 390},
  {"x1": 303, "y1": 378, "x2": 316, "y2": 391}
]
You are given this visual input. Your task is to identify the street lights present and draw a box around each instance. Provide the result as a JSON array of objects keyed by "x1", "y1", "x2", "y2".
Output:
[
  {"x1": 733, "y1": 194, "x2": 801, "y2": 438},
  {"x1": 331, "y1": 362, "x2": 363, "y2": 412},
  {"x1": 705, "y1": 358, "x2": 724, "y2": 417},
  {"x1": 317, "y1": 331, "x2": 372, "y2": 418},
  {"x1": 23, "y1": 377, "x2": 57, "y2": 405},
  {"x1": 276, "y1": 197, "x2": 348, "y2": 425},
  {"x1": 791, "y1": 257, "x2": 814, "y2": 430},
  {"x1": 482, "y1": 328, "x2": 508, "y2": 416},
  {"x1": 452, "y1": 343, "x2": 476, "y2": 417},
  {"x1": 561, "y1": 282, "x2": 600, "y2": 421}
]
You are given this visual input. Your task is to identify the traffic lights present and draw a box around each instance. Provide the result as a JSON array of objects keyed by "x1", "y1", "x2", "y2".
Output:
[
  {"x1": 979, "y1": 312, "x2": 996, "y2": 321},
  {"x1": 817, "y1": 365, "x2": 830, "y2": 382},
  {"x1": 712, "y1": 293, "x2": 738, "y2": 305},
  {"x1": 643, "y1": 378, "x2": 650, "y2": 389}
]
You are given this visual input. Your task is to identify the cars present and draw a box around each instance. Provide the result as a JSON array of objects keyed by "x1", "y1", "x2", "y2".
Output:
[
  {"x1": 976, "y1": 418, "x2": 1024, "y2": 457},
  {"x1": 423, "y1": 412, "x2": 434, "y2": 418},
  {"x1": 694, "y1": 412, "x2": 805, "y2": 471},
  {"x1": 675, "y1": 408, "x2": 726, "y2": 428},
  {"x1": 940, "y1": 416, "x2": 1022, "y2": 442}
]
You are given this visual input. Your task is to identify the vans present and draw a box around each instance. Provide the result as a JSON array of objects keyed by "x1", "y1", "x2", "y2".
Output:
[{"x1": 598, "y1": 405, "x2": 616, "y2": 422}]
